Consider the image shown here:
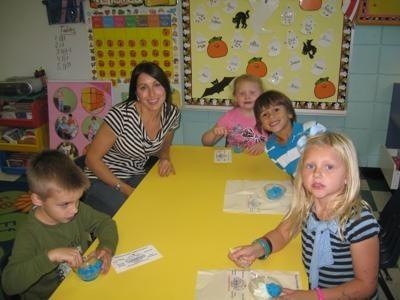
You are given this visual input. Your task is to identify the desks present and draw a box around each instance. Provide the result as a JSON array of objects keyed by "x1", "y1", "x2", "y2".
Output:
[{"x1": 48, "y1": 144, "x2": 309, "y2": 299}]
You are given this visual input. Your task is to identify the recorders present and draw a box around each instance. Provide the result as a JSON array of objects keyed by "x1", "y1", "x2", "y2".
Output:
[{"x1": 0, "y1": 77, "x2": 42, "y2": 95}]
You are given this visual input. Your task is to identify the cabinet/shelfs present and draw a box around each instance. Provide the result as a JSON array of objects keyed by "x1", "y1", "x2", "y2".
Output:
[{"x1": 0, "y1": 89, "x2": 49, "y2": 192}]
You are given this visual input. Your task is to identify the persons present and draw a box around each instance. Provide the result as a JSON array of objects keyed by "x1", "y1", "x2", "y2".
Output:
[
  {"x1": 80, "y1": 62, "x2": 181, "y2": 219},
  {"x1": 1, "y1": 150, "x2": 119, "y2": 300},
  {"x1": 86, "y1": 115, "x2": 100, "y2": 142},
  {"x1": 53, "y1": 88, "x2": 78, "y2": 140},
  {"x1": 253, "y1": 90, "x2": 327, "y2": 181},
  {"x1": 201, "y1": 74, "x2": 269, "y2": 156},
  {"x1": 227, "y1": 132, "x2": 381, "y2": 300}
]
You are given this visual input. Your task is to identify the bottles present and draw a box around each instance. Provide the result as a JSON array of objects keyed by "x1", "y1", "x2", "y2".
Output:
[{"x1": 35, "y1": 66, "x2": 47, "y2": 90}]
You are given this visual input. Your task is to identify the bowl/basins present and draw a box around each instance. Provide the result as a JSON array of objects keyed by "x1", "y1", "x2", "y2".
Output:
[
  {"x1": 249, "y1": 276, "x2": 283, "y2": 299},
  {"x1": 264, "y1": 182, "x2": 285, "y2": 200},
  {"x1": 71, "y1": 256, "x2": 103, "y2": 281},
  {"x1": 231, "y1": 142, "x2": 246, "y2": 153}
]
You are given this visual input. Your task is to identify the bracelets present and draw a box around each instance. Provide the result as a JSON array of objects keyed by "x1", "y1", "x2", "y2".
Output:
[
  {"x1": 114, "y1": 181, "x2": 122, "y2": 192},
  {"x1": 315, "y1": 288, "x2": 324, "y2": 300},
  {"x1": 253, "y1": 237, "x2": 272, "y2": 260}
]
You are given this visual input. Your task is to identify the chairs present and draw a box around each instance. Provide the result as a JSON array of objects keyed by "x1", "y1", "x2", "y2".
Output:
[{"x1": 376, "y1": 182, "x2": 400, "y2": 299}]
